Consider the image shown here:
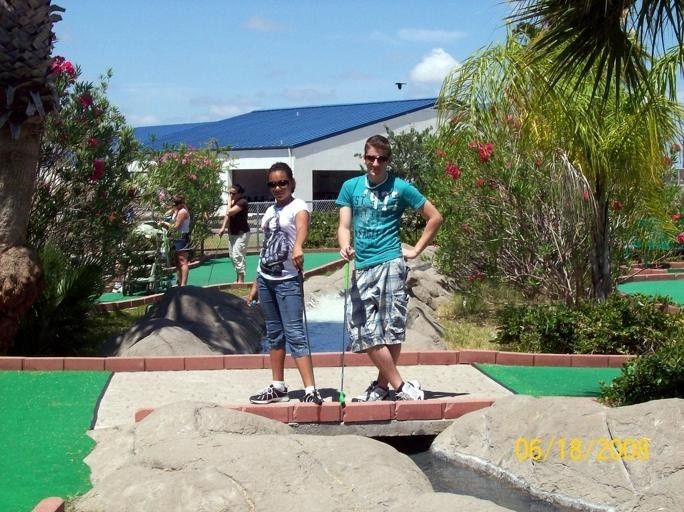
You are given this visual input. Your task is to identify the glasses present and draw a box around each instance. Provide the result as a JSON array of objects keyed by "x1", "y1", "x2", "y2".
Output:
[
  {"x1": 230, "y1": 192, "x2": 237, "y2": 195},
  {"x1": 267, "y1": 180, "x2": 289, "y2": 187},
  {"x1": 366, "y1": 155, "x2": 387, "y2": 162}
]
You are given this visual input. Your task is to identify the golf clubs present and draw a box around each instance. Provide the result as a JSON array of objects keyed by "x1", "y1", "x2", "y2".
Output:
[
  {"x1": 338, "y1": 262, "x2": 349, "y2": 403},
  {"x1": 203, "y1": 232, "x2": 222, "y2": 287},
  {"x1": 298, "y1": 270, "x2": 322, "y2": 405}
]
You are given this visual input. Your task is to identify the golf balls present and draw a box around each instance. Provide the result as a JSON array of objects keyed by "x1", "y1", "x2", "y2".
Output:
[{"x1": 252, "y1": 300, "x2": 256, "y2": 306}]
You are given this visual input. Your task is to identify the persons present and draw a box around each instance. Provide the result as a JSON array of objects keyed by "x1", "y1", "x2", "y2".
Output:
[
  {"x1": 218, "y1": 184, "x2": 251, "y2": 285},
  {"x1": 107, "y1": 191, "x2": 191, "y2": 294},
  {"x1": 334, "y1": 133, "x2": 444, "y2": 400},
  {"x1": 246, "y1": 161, "x2": 324, "y2": 404}
]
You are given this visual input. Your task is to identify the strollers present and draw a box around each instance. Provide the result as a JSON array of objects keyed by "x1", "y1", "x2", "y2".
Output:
[{"x1": 119, "y1": 220, "x2": 172, "y2": 296}]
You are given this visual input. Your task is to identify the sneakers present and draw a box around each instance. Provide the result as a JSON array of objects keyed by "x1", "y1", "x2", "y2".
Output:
[
  {"x1": 250, "y1": 384, "x2": 289, "y2": 404},
  {"x1": 303, "y1": 388, "x2": 324, "y2": 404},
  {"x1": 352, "y1": 381, "x2": 392, "y2": 402},
  {"x1": 394, "y1": 379, "x2": 424, "y2": 401}
]
